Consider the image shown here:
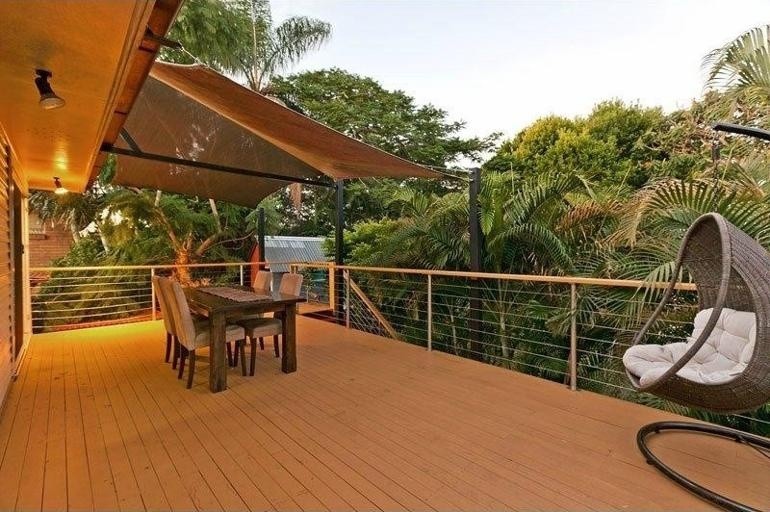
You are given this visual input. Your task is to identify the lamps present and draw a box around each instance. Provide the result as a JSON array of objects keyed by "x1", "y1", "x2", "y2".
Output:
[
  {"x1": 52, "y1": 176, "x2": 68, "y2": 197},
  {"x1": 33, "y1": 67, "x2": 66, "y2": 113}
]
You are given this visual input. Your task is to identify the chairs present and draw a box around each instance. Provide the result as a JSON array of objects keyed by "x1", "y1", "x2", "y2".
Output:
[{"x1": 151, "y1": 270, "x2": 307, "y2": 395}]
